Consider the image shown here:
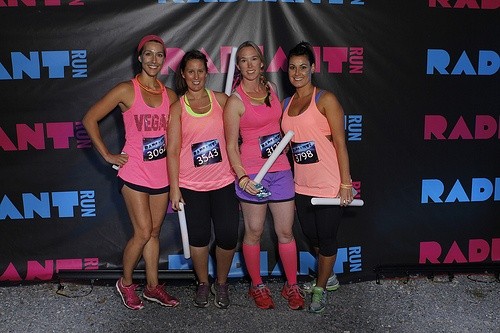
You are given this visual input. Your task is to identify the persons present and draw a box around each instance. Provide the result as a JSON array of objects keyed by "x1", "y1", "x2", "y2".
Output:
[
  {"x1": 166, "y1": 49, "x2": 240, "y2": 308},
  {"x1": 222, "y1": 40, "x2": 305, "y2": 309},
  {"x1": 281, "y1": 42, "x2": 354, "y2": 313},
  {"x1": 81, "y1": 35, "x2": 179, "y2": 310}
]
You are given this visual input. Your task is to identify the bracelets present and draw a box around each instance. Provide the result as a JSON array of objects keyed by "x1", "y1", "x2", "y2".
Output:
[
  {"x1": 238, "y1": 175, "x2": 250, "y2": 191},
  {"x1": 340, "y1": 186, "x2": 351, "y2": 189}
]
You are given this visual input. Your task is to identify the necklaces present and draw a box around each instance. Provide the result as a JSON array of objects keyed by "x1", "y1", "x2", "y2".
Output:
[
  {"x1": 136, "y1": 74, "x2": 164, "y2": 94},
  {"x1": 184, "y1": 93, "x2": 212, "y2": 109},
  {"x1": 340, "y1": 183, "x2": 352, "y2": 186},
  {"x1": 240, "y1": 80, "x2": 267, "y2": 100}
]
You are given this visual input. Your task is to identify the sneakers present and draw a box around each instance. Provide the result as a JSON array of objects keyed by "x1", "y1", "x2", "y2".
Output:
[
  {"x1": 281, "y1": 282, "x2": 305, "y2": 309},
  {"x1": 116, "y1": 278, "x2": 144, "y2": 310},
  {"x1": 142, "y1": 282, "x2": 181, "y2": 307},
  {"x1": 307, "y1": 285, "x2": 327, "y2": 312},
  {"x1": 193, "y1": 280, "x2": 210, "y2": 307},
  {"x1": 211, "y1": 279, "x2": 230, "y2": 308},
  {"x1": 299, "y1": 271, "x2": 340, "y2": 292},
  {"x1": 248, "y1": 281, "x2": 275, "y2": 309}
]
